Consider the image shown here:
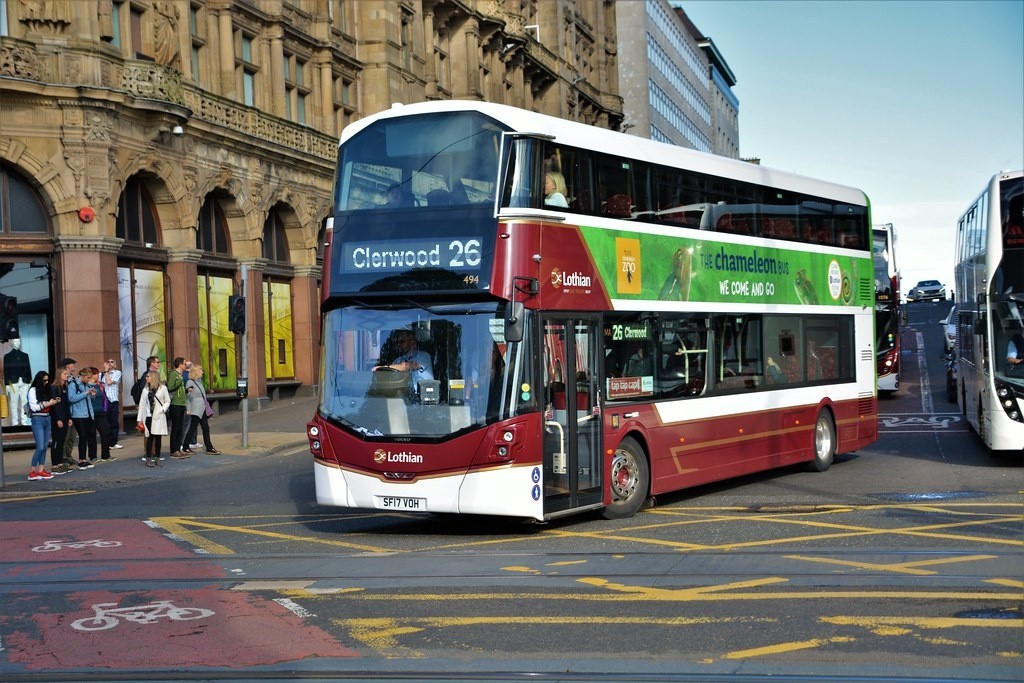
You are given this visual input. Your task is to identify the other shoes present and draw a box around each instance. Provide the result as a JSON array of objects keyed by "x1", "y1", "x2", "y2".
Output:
[
  {"x1": 100, "y1": 456, "x2": 118, "y2": 462},
  {"x1": 155, "y1": 457, "x2": 163, "y2": 467},
  {"x1": 146, "y1": 457, "x2": 156, "y2": 467},
  {"x1": 90, "y1": 458, "x2": 101, "y2": 464},
  {"x1": 109, "y1": 444, "x2": 124, "y2": 450},
  {"x1": 159, "y1": 457, "x2": 165, "y2": 461},
  {"x1": 61, "y1": 459, "x2": 78, "y2": 466},
  {"x1": 142, "y1": 457, "x2": 155, "y2": 461}
]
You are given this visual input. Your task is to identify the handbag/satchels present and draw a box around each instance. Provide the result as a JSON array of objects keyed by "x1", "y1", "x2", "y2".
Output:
[
  {"x1": 23, "y1": 402, "x2": 33, "y2": 418},
  {"x1": 205, "y1": 400, "x2": 213, "y2": 418}
]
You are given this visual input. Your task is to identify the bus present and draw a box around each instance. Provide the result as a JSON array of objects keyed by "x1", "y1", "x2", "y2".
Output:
[
  {"x1": 305, "y1": 99, "x2": 908, "y2": 522},
  {"x1": 955, "y1": 170, "x2": 1024, "y2": 451}
]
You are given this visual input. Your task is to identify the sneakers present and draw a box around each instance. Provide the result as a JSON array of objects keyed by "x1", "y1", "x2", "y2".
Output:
[
  {"x1": 78, "y1": 462, "x2": 95, "y2": 470},
  {"x1": 170, "y1": 451, "x2": 191, "y2": 459},
  {"x1": 206, "y1": 448, "x2": 221, "y2": 455},
  {"x1": 182, "y1": 448, "x2": 196, "y2": 455},
  {"x1": 50, "y1": 464, "x2": 73, "y2": 475},
  {"x1": 27, "y1": 471, "x2": 55, "y2": 480}
]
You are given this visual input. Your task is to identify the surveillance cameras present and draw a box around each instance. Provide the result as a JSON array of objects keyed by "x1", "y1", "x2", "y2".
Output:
[{"x1": 173, "y1": 126, "x2": 183, "y2": 136}]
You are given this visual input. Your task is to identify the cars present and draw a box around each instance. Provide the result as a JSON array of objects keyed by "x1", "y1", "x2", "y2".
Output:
[
  {"x1": 904, "y1": 280, "x2": 946, "y2": 303},
  {"x1": 938, "y1": 306, "x2": 956, "y2": 404}
]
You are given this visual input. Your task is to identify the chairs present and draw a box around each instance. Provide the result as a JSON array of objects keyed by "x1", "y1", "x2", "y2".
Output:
[
  {"x1": 688, "y1": 338, "x2": 837, "y2": 394},
  {"x1": 607, "y1": 194, "x2": 830, "y2": 244}
]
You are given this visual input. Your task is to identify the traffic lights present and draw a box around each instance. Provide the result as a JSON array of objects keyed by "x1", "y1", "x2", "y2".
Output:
[{"x1": 228, "y1": 296, "x2": 245, "y2": 334}]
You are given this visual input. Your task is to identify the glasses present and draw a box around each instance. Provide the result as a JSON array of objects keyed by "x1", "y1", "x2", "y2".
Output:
[
  {"x1": 107, "y1": 361, "x2": 114, "y2": 363},
  {"x1": 154, "y1": 362, "x2": 160, "y2": 364},
  {"x1": 43, "y1": 380, "x2": 49, "y2": 382}
]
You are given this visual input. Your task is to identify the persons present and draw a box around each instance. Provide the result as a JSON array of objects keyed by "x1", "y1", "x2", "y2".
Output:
[
  {"x1": 27, "y1": 371, "x2": 61, "y2": 480},
  {"x1": 137, "y1": 371, "x2": 171, "y2": 468},
  {"x1": 529, "y1": 170, "x2": 570, "y2": 208},
  {"x1": 87, "y1": 366, "x2": 117, "y2": 464},
  {"x1": 2, "y1": 331, "x2": 32, "y2": 425},
  {"x1": 98, "y1": 358, "x2": 124, "y2": 449},
  {"x1": 62, "y1": 357, "x2": 78, "y2": 466},
  {"x1": 168, "y1": 357, "x2": 221, "y2": 458},
  {"x1": 767, "y1": 350, "x2": 788, "y2": 385},
  {"x1": 665, "y1": 323, "x2": 699, "y2": 374},
  {"x1": 385, "y1": 183, "x2": 412, "y2": 208},
  {"x1": 606, "y1": 339, "x2": 682, "y2": 400},
  {"x1": 141, "y1": 356, "x2": 166, "y2": 461},
  {"x1": 51, "y1": 369, "x2": 74, "y2": 475},
  {"x1": 68, "y1": 368, "x2": 97, "y2": 470},
  {"x1": 370, "y1": 330, "x2": 435, "y2": 396}
]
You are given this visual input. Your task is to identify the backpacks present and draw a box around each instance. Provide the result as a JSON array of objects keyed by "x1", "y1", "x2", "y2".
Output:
[{"x1": 130, "y1": 379, "x2": 141, "y2": 404}]
